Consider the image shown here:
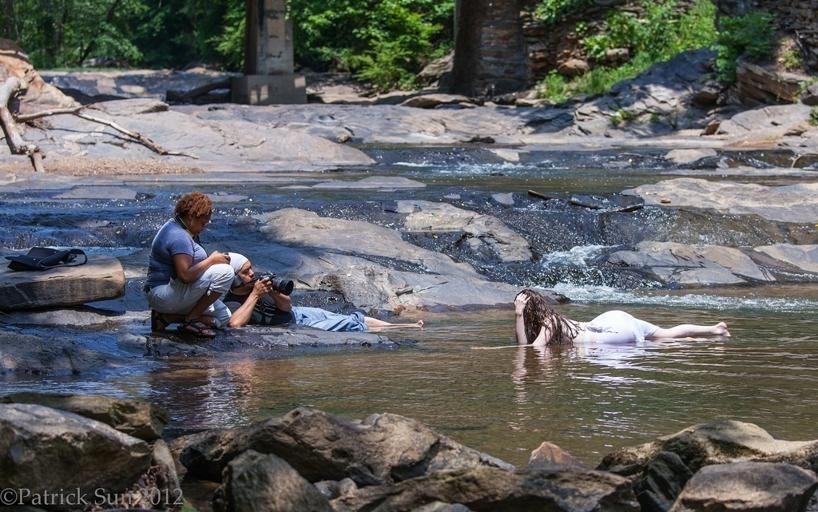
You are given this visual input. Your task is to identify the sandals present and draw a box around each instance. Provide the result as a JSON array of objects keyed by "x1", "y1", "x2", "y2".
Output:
[
  {"x1": 150, "y1": 309, "x2": 169, "y2": 332},
  {"x1": 177, "y1": 320, "x2": 216, "y2": 339}
]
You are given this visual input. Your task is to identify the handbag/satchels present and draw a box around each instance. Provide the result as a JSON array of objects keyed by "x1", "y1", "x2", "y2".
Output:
[{"x1": 6, "y1": 245, "x2": 88, "y2": 271}]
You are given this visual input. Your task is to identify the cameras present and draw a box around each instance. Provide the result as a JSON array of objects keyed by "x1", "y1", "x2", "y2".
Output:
[{"x1": 252, "y1": 272, "x2": 294, "y2": 296}]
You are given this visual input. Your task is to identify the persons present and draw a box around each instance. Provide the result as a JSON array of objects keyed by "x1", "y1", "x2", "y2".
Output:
[
  {"x1": 225, "y1": 250, "x2": 424, "y2": 332},
  {"x1": 514, "y1": 289, "x2": 730, "y2": 348},
  {"x1": 144, "y1": 192, "x2": 235, "y2": 338}
]
